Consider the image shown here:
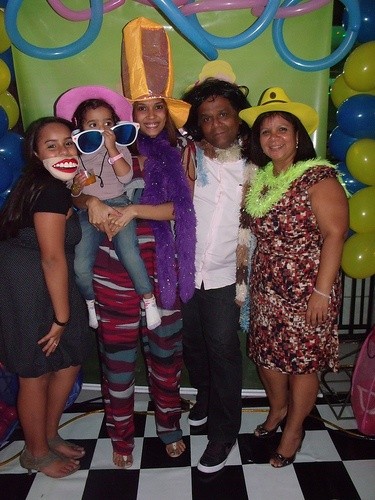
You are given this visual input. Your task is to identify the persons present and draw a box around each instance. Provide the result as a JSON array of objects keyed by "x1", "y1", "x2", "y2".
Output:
[{"x1": 0, "y1": 75, "x2": 349, "y2": 476}]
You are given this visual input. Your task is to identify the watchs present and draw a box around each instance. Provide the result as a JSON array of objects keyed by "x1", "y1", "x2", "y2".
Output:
[{"x1": 108, "y1": 153, "x2": 124, "y2": 165}]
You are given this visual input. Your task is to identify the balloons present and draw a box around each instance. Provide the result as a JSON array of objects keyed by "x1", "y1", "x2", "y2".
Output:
[{"x1": 0, "y1": 0, "x2": 375, "y2": 279}]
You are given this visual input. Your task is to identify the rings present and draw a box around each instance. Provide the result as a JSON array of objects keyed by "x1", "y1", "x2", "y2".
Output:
[{"x1": 54, "y1": 341, "x2": 57, "y2": 346}]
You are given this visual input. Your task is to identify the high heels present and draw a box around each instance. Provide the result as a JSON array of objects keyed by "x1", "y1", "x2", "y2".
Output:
[
  {"x1": 253, "y1": 415, "x2": 289, "y2": 441},
  {"x1": 19, "y1": 444, "x2": 81, "y2": 479},
  {"x1": 48, "y1": 435, "x2": 82, "y2": 460},
  {"x1": 270, "y1": 429, "x2": 306, "y2": 469}
]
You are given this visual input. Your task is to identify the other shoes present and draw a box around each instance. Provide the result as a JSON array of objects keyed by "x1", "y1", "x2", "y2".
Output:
[
  {"x1": 197, "y1": 439, "x2": 237, "y2": 473},
  {"x1": 187, "y1": 407, "x2": 209, "y2": 427}
]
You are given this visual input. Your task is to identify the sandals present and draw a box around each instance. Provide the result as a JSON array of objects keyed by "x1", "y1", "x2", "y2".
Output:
[
  {"x1": 165, "y1": 442, "x2": 184, "y2": 458},
  {"x1": 113, "y1": 454, "x2": 133, "y2": 469}
]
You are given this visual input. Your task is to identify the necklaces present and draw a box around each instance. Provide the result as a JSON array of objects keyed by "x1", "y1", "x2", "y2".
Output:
[
  {"x1": 77, "y1": 150, "x2": 107, "y2": 188},
  {"x1": 197, "y1": 137, "x2": 242, "y2": 162},
  {"x1": 135, "y1": 129, "x2": 196, "y2": 307},
  {"x1": 245, "y1": 159, "x2": 352, "y2": 218}
]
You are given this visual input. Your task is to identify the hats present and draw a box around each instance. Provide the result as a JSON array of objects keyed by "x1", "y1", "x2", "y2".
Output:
[
  {"x1": 238, "y1": 86, "x2": 321, "y2": 136},
  {"x1": 54, "y1": 85, "x2": 134, "y2": 125},
  {"x1": 120, "y1": 16, "x2": 193, "y2": 129}
]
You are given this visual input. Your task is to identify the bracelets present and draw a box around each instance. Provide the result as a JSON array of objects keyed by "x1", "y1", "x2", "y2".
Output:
[
  {"x1": 53, "y1": 314, "x2": 71, "y2": 326},
  {"x1": 71, "y1": 190, "x2": 82, "y2": 197},
  {"x1": 313, "y1": 287, "x2": 331, "y2": 299}
]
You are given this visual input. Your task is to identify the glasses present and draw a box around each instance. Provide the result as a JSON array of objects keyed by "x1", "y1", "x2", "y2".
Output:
[{"x1": 71, "y1": 121, "x2": 141, "y2": 155}]
